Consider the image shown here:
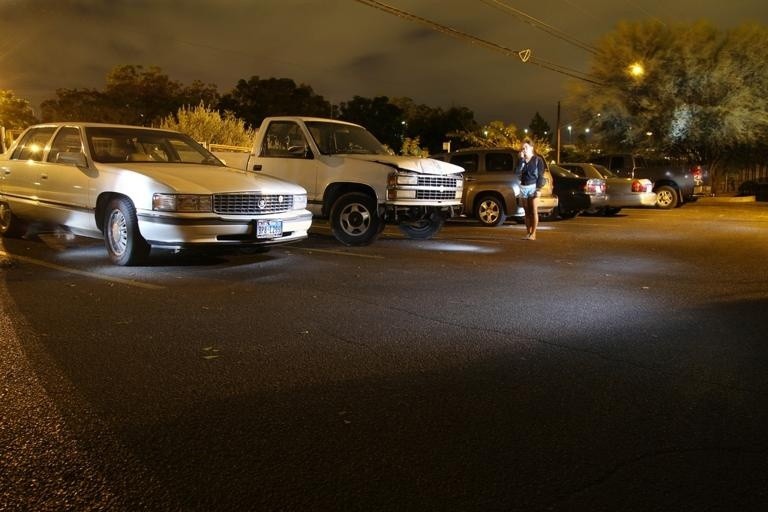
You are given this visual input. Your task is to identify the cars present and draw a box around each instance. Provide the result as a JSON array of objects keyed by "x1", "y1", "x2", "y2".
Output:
[
  {"x1": 547, "y1": 154, "x2": 714, "y2": 220},
  {"x1": 0, "y1": 121, "x2": 314, "y2": 266}
]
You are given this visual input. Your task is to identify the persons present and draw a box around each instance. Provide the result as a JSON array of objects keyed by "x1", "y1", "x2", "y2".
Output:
[{"x1": 512, "y1": 139, "x2": 545, "y2": 242}]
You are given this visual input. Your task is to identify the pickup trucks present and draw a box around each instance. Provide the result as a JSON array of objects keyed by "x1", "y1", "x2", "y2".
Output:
[{"x1": 126, "y1": 115, "x2": 466, "y2": 246}]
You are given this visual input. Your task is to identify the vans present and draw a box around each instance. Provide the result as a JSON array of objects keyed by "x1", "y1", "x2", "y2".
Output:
[{"x1": 427, "y1": 147, "x2": 559, "y2": 227}]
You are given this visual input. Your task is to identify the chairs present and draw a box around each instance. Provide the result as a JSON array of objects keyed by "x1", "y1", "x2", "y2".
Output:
[{"x1": 126, "y1": 142, "x2": 149, "y2": 161}]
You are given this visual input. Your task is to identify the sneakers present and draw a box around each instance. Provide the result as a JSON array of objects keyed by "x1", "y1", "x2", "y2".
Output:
[{"x1": 521, "y1": 232, "x2": 538, "y2": 241}]
